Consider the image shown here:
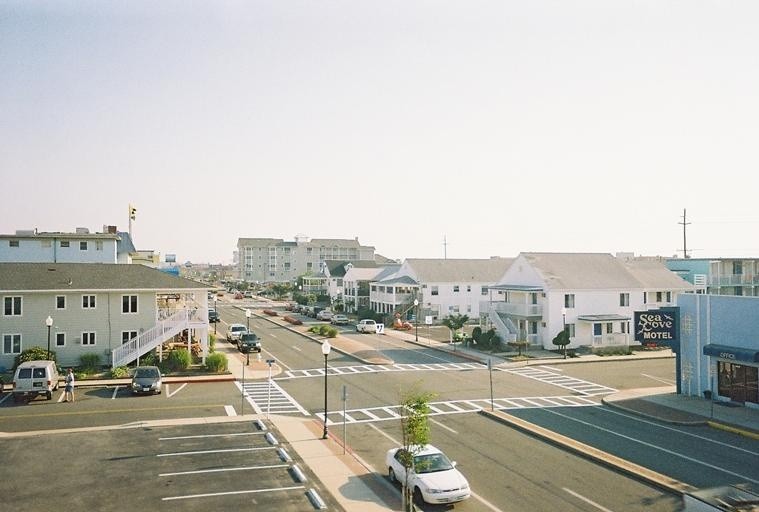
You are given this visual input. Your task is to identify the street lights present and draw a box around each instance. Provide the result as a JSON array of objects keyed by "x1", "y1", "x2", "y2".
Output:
[
  {"x1": 337, "y1": 289, "x2": 340, "y2": 314},
  {"x1": 293, "y1": 282, "x2": 302, "y2": 302},
  {"x1": 321, "y1": 339, "x2": 330, "y2": 438},
  {"x1": 46, "y1": 316, "x2": 53, "y2": 360},
  {"x1": 414, "y1": 298, "x2": 419, "y2": 341},
  {"x1": 562, "y1": 306, "x2": 567, "y2": 360},
  {"x1": 246, "y1": 308, "x2": 251, "y2": 366}
]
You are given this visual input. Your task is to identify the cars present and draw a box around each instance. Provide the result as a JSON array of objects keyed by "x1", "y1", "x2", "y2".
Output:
[
  {"x1": 355, "y1": 319, "x2": 377, "y2": 334},
  {"x1": 286, "y1": 303, "x2": 314, "y2": 315},
  {"x1": 385, "y1": 444, "x2": 471, "y2": 507},
  {"x1": 235, "y1": 291, "x2": 253, "y2": 299},
  {"x1": 307, "y1": 306, "x2": 334, "y2": 321},
  {"x1": 208, "y1": 289, "x2": 228, "y2": 299},
  {"x1": 130, "y1": 366, "x2": 162, "y2": 396},
  {"x1": 209, "y1": 309, "x2": 221, "y2": 323},
  {"x1": 330, "y1": 314, "x2": 348, "y2": 325},
  {"x1": 226, "y1": 324, "x2": 261, "y2": 353}
]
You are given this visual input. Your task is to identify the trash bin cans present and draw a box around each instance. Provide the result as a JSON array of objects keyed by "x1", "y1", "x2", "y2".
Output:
[
  {"x1": 703, "y1": 390, "x2": 711, "y2": 400},
  {"x1": 464, "y1": 338, "x2": 473, "y2": 348}
]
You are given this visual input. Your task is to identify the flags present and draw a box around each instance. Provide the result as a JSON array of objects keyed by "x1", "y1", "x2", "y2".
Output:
[{"x1": 128, "y1": 203, "x2": 138, "y2": 220}]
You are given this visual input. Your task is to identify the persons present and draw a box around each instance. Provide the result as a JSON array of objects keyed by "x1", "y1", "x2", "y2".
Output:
[{"x1": 63, "y1": 368, "x2": 75, "y2": 403}]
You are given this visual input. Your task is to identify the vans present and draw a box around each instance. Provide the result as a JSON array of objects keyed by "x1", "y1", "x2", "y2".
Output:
[{"x1": 12, "y1": 360, "x2": 62, "y2": 401}]
